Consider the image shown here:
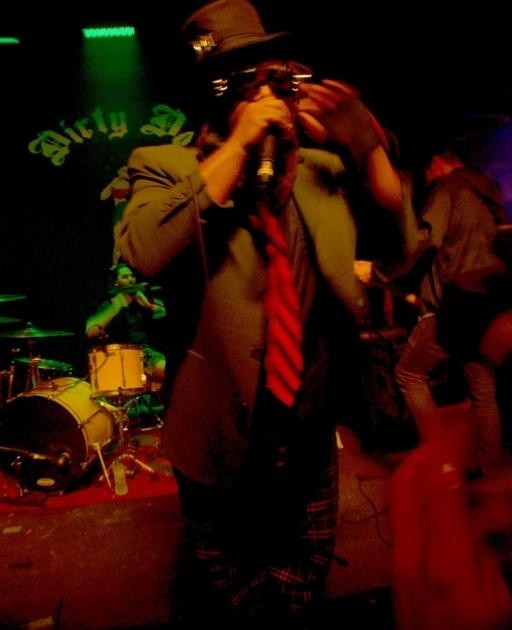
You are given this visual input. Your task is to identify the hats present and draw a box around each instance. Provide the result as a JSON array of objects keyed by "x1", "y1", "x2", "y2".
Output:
[{"x1": 179, "y1": 1, "x2": 291, "y2": 82}]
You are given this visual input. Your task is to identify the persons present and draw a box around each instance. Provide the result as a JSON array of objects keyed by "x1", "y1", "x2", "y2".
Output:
[
  {"x1": 110, "y1": 1, "x2": 419, "y2": 630},
  {"x1": 80, "y1": 264, "x2": 170, "y2": 427},
  {"x1": 387, "y1": 139, "x2": 510, "y2": 481}
]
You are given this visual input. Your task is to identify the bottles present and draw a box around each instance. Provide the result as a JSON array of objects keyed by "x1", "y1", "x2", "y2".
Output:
[{"x1": 111, "y1": 458, "x2": 129, "y2": 496}]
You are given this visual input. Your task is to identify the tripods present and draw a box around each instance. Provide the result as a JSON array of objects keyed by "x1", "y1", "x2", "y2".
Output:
[{"x1": 94, "y1": 397, "x2": 165, "y2": 487}]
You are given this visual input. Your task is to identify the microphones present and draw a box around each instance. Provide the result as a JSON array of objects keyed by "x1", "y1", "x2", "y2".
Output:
[{"x1": 255, "y1": 132, "x2": 281, "y2": 185}]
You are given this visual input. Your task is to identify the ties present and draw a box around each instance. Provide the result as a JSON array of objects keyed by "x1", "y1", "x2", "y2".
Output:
[{"x1": 246, "y1": 200, "x2": 303, "y2": 409}]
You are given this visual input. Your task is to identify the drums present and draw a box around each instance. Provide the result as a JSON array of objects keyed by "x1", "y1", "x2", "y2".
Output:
[
  {"x1": 1, "y1": 376, "x2": 124, "y2": 494},
  {"x1": 88, "y1": 342, "x2": 148, "y2": 400}
]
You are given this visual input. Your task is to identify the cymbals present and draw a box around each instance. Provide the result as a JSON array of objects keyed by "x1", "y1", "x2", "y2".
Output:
[
  {"x1": 0, "y1": 293, "x2": 26, "y2": 304},
  {"x1": 4, "y1": 325, "x2": 74, "y2": 339},
  {"x1": 14, "y1": 355, "x2": 78, "y2": 375},
  {"x1": 1, "y1": 315, "x2": 22, "y2": 326}
]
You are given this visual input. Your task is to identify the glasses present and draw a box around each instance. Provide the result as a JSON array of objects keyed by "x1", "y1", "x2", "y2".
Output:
[{"x1": 213, "y1": 65, "x2": 313, "y2": 97}]
